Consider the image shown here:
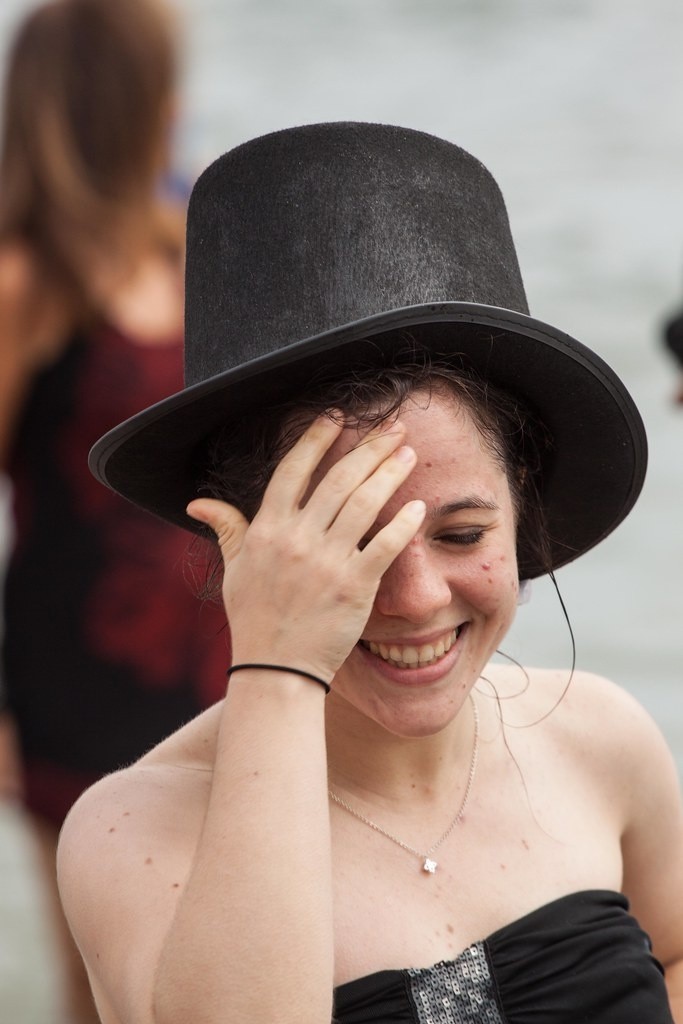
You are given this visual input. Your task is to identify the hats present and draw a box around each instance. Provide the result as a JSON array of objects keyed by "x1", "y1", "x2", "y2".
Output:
[{"x1": 87, "y1": 121, "x2": 648, "y2": 582}]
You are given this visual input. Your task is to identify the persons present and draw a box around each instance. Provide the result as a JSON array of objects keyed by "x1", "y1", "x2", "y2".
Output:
[
  {"x1": 0, "y1": 1, "x2": 232, "y2": 826},
  {"x1": 55, "y1": 121, "x2": 683, "y2": 1024}
]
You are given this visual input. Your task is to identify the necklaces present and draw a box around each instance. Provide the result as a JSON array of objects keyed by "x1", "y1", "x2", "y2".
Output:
[{"x1": 329, "y1": 663, "x2": 479, "y2": 880}]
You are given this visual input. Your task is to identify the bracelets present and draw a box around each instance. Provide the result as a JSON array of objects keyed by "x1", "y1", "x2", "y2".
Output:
[{"x1": 222, "y1": 661, "x2": 329, "y2": 695}]
